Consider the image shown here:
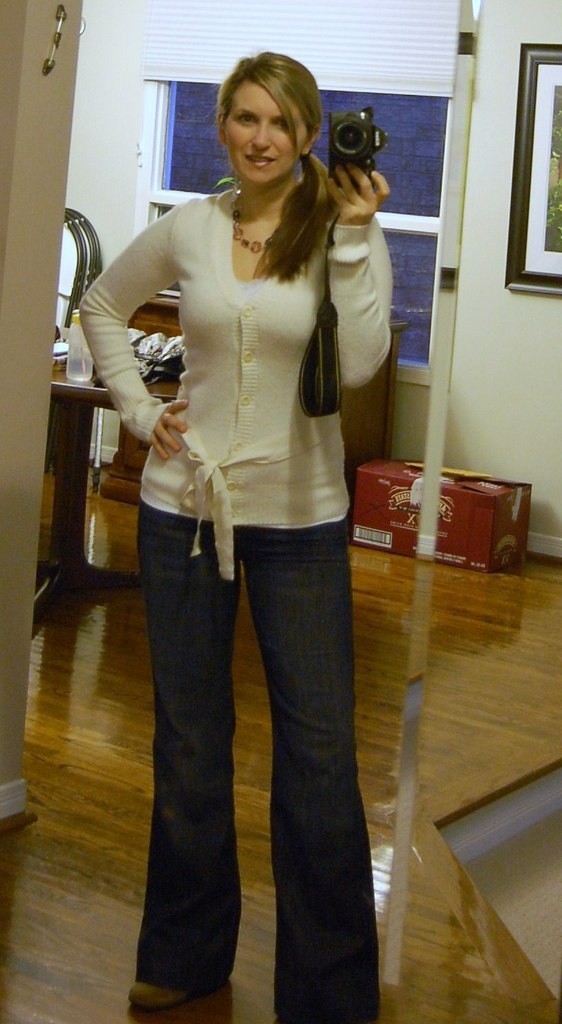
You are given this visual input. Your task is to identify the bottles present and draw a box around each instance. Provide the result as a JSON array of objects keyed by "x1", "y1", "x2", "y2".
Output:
[{"x1": 66, "y1": 314, "x2": 93, "y2": 381}]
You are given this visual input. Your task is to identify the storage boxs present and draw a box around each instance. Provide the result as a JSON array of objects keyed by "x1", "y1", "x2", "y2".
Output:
[{"x1": 348, "y1": 459, "x2": 532, "y2": 574}]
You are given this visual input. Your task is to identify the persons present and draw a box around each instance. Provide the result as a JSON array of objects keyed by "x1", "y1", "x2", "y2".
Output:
[{"x1": 80, "y1": 51, "x2": 393, "y2": 1023}]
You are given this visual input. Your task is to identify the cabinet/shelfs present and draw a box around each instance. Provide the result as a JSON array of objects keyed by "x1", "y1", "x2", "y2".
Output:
[{"x1": 96, "y1": 294, "x2": 409, "y2": 506}]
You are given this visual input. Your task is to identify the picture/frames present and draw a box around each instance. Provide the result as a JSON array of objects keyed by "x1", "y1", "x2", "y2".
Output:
[{"x1": 505, "y1": 43, "x2": 562, "y2": 296}]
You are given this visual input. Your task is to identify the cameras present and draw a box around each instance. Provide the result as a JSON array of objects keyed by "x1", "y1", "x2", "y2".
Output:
[{"x1": 330, "y1": 107, "x2": 391, "y2": 187}]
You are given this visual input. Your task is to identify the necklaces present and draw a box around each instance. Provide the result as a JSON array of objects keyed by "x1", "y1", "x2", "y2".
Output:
[{"x1": 232, "y1": 189, "x2": 275, "y2": 253}]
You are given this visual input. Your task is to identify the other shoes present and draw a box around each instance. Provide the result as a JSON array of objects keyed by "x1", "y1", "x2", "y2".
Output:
[{"x1": 129, "y1": 982, "x2": 189, "y2": 1011}]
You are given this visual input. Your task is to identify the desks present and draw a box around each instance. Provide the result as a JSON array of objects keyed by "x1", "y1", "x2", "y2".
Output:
[{"x1": 33, "y1": 365, "x2": 181, "y2": 620}]
